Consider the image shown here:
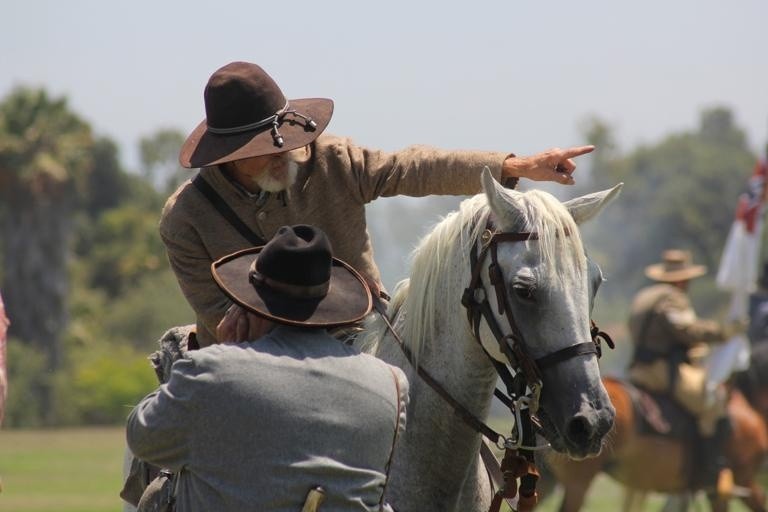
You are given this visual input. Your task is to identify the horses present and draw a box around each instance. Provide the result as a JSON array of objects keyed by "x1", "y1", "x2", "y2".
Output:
[
  {"x1": 528, "y1": 372, "x2": 768, "y2": 512},
  {"x1": 118, "y1": 165, "x2": 624, "y2": 511}
]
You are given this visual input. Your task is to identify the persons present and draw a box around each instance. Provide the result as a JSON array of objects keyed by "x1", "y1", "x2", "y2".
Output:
[
  {"x1": 621, "y1": 248, "x2": 754, "y2": 499},
  {"x1": 157, "y1": 61, "x2": 597, "y2": 349},
  {"x1": 126, "y1": 221, "x2": 413, "y2": 512}
]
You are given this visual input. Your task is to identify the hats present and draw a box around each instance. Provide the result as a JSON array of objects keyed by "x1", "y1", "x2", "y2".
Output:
[
  {"x1": 645, "y1": 250, "x2": 707, "y2": 282},
  {"x1": 210, "y1": 224, "x2": 373, "y2": 329},
  {"x1": 178, "y1": 61, "x2": 334, "y2": 170}
]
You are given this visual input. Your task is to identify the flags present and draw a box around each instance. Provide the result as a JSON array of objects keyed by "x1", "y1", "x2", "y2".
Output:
[{"x1": 713, "y1": 144, "x2": 768, "y2": 298}]
